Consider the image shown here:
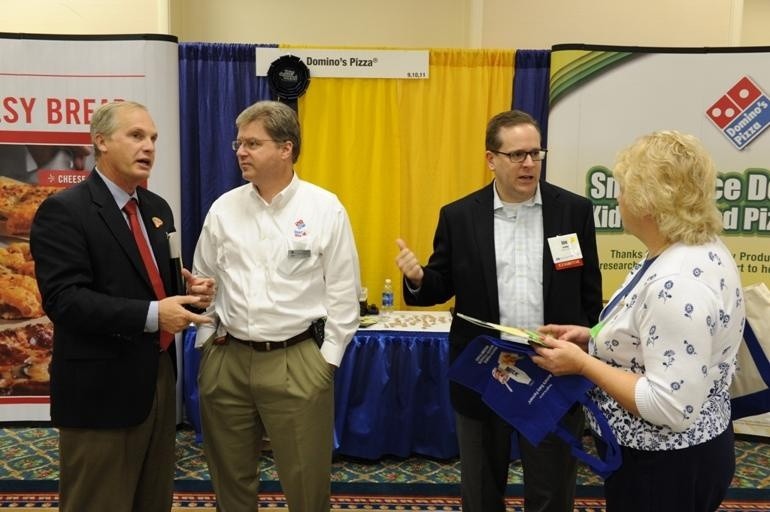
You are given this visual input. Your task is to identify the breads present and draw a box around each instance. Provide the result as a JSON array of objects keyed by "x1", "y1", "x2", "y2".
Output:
[{"x1": 0, "y1": 180, "x2": 66, "y2": 321}]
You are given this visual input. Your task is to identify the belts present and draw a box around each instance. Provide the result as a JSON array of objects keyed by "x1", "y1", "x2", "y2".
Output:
[{"x1": 226, "y1": 324, "x2": 313, "y2": 353}]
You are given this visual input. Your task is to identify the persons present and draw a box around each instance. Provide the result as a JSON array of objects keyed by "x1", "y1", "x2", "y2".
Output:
[
  {"x1": 29, "y1": 100, "x2": 215, "y2": 512},
  {"x1": 187, "y1": 101, "x2": 360, "y2": 512},
  {"x1": 394, "y1": 109, "x2": 604, "y2": 511},
  {"x1": 528, "y1": 130, "x2": 745, "y2": 512}
]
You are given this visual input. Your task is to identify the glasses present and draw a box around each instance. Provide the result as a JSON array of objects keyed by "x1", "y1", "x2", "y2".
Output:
[
  {"x1": 231, "y1": 137, "x2": 286, "y2": 151},
  {"x1": 490, "y1": 149, "x2": 548, "y2": 162}
]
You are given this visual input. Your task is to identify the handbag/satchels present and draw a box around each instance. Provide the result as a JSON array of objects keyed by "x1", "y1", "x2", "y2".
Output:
[
  {"x1": 728, "y1": 281, "x2": 770, "y2": 422},
  {"x1": 445, "y1": 335, "x2": 623, "y2": 473},
  {"x1": 313, "y1": 318, "x2": 325, "y2": 348}
]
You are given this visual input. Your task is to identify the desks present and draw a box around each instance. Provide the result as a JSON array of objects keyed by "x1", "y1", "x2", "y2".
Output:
[{"x1": 185, "y1": 308, "x2": 456, "y2": 465}]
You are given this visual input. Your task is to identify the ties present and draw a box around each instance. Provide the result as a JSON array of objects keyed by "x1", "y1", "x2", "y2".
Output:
[{"x1": 122, "y1": 199, "x2": 174, "y2": 352}]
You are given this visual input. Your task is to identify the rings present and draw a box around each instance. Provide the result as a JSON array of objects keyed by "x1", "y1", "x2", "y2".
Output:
[{"x1": 206, "y1": 295, "x2": 209, "y2": 301}]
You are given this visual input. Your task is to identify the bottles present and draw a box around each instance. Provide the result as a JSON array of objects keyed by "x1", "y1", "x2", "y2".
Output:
[{"x1": 381, "y1": 279, "x2": 394, "y2": 321}]
